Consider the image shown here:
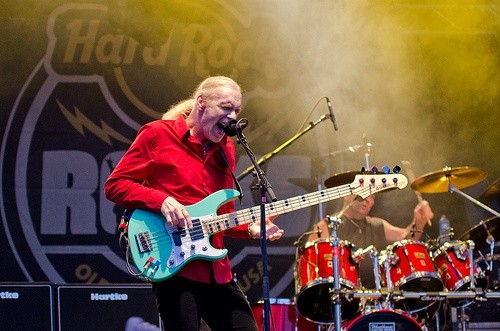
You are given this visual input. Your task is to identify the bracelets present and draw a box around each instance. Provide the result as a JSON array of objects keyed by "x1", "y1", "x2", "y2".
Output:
[{"x1": 248, "y1": 221, "x2": 256, "y2": 240}]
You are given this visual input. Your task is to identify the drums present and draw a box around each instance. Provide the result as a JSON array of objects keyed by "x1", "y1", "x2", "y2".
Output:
[
  {"x1": 429, "y1": 240, "x2": 488, "y2": 308},
  {"x1": 342, "y1": 309, "x2": 424, "y2": 331},
  {"x1": 253, "y1": 297, "x2": 319, "y2": 331},
  {"x1": 294, "y1": 237, "x2": 360, "y2": 326},
  {"x1": 378, "y1": 238, "x2": 443, "y2": 314}
]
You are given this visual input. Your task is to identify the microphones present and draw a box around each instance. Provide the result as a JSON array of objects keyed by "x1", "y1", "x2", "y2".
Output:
[
  {"x1": 364, "y1": 137, "x2": 371, "y2": 152},
  {"x1": 326, "y1": 97, "x2": 338, "y2": 131},
  {"x1": 224, "y1": 119, "x2": 247, "y2": 136}
]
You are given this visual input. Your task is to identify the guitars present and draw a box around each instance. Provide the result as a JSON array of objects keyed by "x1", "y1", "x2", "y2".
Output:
[{"x1": 127, "y1": 166, "x2": 408, "y2": 284}]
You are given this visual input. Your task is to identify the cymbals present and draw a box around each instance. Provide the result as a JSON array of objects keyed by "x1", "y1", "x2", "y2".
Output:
[
  {"x1": 324, "y1": 170, "x2": 398, "y2": 194},
  {"x1": 473, "y1": 177, "x2": 500, "y2": 207},
  {"x1": 457, "y1": 216, "x2": 500, "y2": 250},
  {"x1": 410, "y1": 166, "x2": 489, "y2": 193}
]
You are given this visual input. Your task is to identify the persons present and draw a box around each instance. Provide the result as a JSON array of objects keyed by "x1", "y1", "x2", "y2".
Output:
[
  {"x1": 104, "y1": 76, "x2": 284, "y2": 331},
  {"x1": 308, "y1": 195, "x2": 435, "y2": 289}
]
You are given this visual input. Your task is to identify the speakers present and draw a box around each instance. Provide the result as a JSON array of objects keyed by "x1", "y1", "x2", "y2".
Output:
[
  {"x1": 0, "y1": 282, "x2": 55, "y2": 331},
  {"x1": 53, "y1": 283, "x2": 161, "y2": 331}
]
